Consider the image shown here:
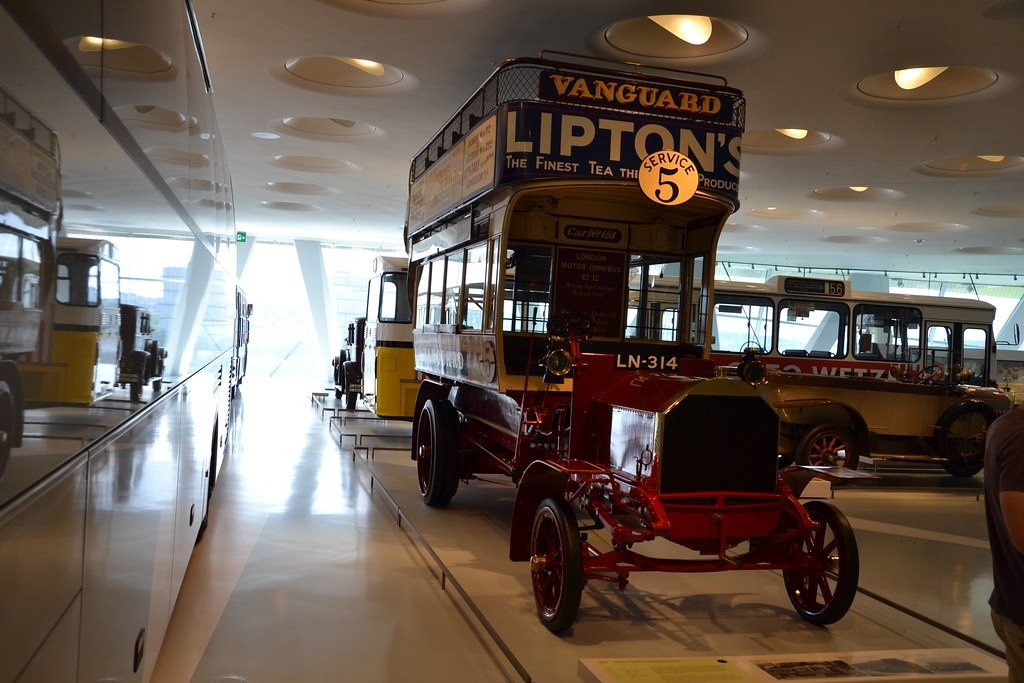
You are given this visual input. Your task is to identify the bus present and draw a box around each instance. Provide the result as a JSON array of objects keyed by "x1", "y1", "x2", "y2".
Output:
[
  {"x1": 1, "y1": 82, "x2": 64, "y2": 472},
  {"x1": 16, "y1": 236, "x2": 122, "y2": 410},
  {"x1": 0, "y1": 0, "x2": 255, "y2": 680},
  {"x1": 363, "y1": 255, "x2": 999, "y2": 477}
]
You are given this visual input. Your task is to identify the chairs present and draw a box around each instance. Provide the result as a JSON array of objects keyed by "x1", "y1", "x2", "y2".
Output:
[
  {"x1": 859, "y1": 353, "x2": 884, "y2": 361},
  {"x1": 809, "y1": 349, "x2": 835, "y2": 358},
  {"x1": 781, "y1": 349, "x2": 809, "y2": 358}
]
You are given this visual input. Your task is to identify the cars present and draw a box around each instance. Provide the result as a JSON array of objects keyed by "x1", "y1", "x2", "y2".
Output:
[
  {"x1": 119, "y1": 304, "x2": 170, "y2": 405},
  {"x1": 331, "y1": 318, "x2": 363, "y2": 410},
  {"x1": 402, "y1": 49, "x2": 859, "y2": 639}
]
